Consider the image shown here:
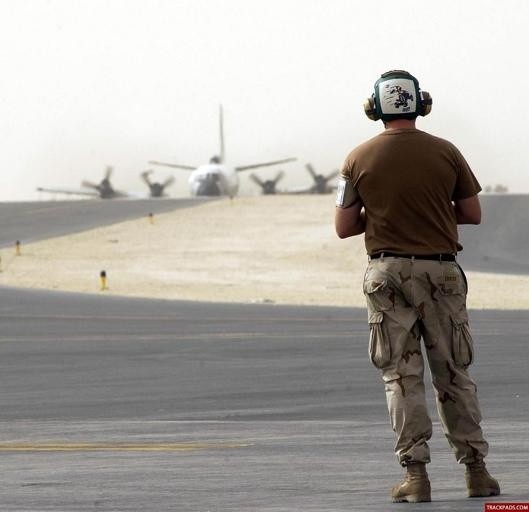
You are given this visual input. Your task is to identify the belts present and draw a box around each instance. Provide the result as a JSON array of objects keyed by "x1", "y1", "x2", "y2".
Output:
[{"x1": 369, "y1": 251, "x2": 456, "y2": 263}]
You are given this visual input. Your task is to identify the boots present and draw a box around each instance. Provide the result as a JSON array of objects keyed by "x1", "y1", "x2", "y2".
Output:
[
  {"x1": 390, "y1": 460, "x2": 430, "y2": 501},
  {"x1": 465, "y1": 458, "x2": 500, "y2": 497}
]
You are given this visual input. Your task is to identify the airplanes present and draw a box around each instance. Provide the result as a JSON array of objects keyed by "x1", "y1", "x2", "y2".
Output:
[{"x1": 32, "y1": 102, "x2": 353, "y2": 206}]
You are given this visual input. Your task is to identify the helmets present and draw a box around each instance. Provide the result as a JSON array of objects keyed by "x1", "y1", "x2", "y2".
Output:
[{"x1": 363, "y1": 69, "x2": 433, "y2": 120}]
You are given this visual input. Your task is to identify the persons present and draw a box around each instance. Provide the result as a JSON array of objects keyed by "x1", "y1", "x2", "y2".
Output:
[{"x1": 334, "y1": 70, "x2": 500, "y2": 503}]
